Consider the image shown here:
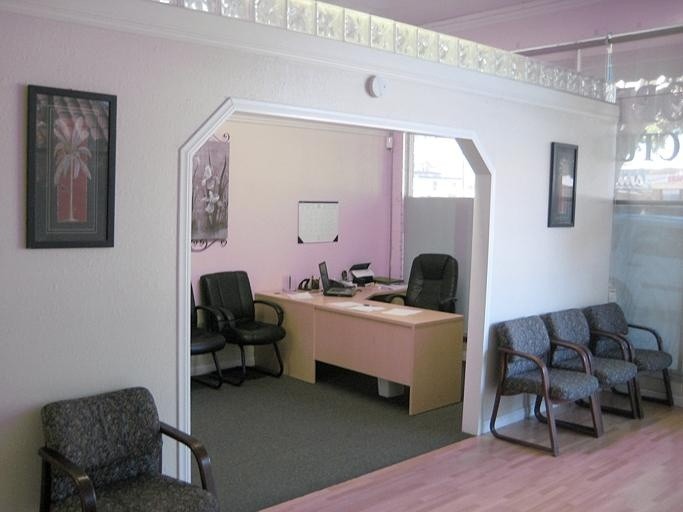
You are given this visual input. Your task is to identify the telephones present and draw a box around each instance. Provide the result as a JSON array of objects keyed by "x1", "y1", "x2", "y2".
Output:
[{"x1": 342, "y1": 271, "x2": 347, "y2": 280}]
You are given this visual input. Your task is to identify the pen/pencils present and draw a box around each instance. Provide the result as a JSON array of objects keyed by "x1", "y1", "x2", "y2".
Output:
[{"x1": 312, "y1": 276, "x2": 320, "y2": 289}]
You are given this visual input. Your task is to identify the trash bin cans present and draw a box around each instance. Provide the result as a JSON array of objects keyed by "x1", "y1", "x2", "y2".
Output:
[{"x1": 378, "y1": 378, "x2": 405, "y2": 397}]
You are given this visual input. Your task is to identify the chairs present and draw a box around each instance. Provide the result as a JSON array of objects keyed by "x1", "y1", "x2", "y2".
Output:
[
  {"x1": 385, "y1": 252, "x2": 459, "y2": 312},
  {"x1": 35, "y1": 385, "x2": 218, "y2": 512},
  {"x1": 488, "y1": 303, "x2": 676, "y2": 456},
  {"x1": 188, "y1": 268, "x2": 286, "y2": 390}
]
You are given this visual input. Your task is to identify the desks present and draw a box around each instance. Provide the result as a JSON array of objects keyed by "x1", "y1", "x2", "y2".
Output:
[{"x1": 254, "y1": 279, "x2": 463, "y2": 416}]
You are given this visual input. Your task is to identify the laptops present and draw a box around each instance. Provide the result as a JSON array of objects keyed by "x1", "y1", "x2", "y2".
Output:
[{"x1": 319, "y1": 261, "x2": 356, "y2": 296}]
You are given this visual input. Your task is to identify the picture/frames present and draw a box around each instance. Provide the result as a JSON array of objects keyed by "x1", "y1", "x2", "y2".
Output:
[
  {"x1": 28, "y1": 85, "x2": 117, "y2": 250},
  {"x1": 547, "y1": 142, "x2": 578, "y2": 227}
]
